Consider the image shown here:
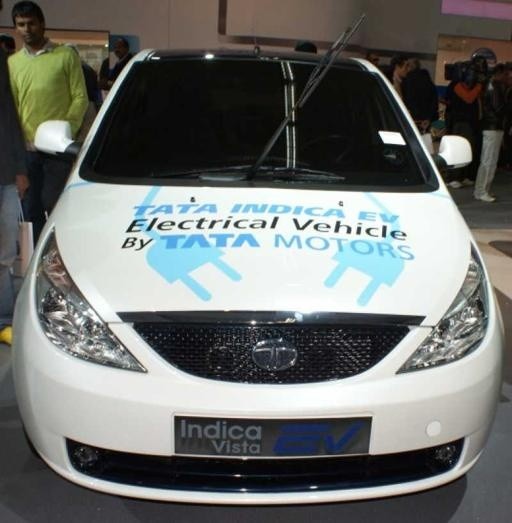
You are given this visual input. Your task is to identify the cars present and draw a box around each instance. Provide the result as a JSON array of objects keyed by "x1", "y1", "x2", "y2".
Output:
[{"x1": 12, "y1": 48, "x2": 505, "y2": 510}]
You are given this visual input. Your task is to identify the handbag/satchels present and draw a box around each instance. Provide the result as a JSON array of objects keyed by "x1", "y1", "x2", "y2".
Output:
[{"x1": 12, "y1": 195, "x2": 34, "y2": 278}]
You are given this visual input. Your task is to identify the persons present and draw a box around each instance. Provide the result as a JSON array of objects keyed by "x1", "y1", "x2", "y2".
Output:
[
  {"x1": 0, "y1": 49, "x2": 31, "y2": 346},
  {"x1": 1, "y1": 31, "x2": 17, "y2": 57},
  {"x1": 65, "y1": 42, "x2": 105, "y2": 112},
  {"x1": 99, "y1": 36, "x2": 138, "y2": 103},
  {"x1": 6, "y1": 0, "x2": 90, "y2": 250},
  {"x1": 294, "y1": 40, "x2": 511, "y2": 203}
]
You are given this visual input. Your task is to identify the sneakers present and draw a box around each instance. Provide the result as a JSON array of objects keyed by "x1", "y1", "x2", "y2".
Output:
[
  {"x1": 474, "y1": 193, "x2": 496, "y2": 202},
  {"x1": 0, "y1": 325, "x2": 12, "y2": 346},
  {"x1": 445, "y1": 178, "x2": 475, "y2": 188}
]
